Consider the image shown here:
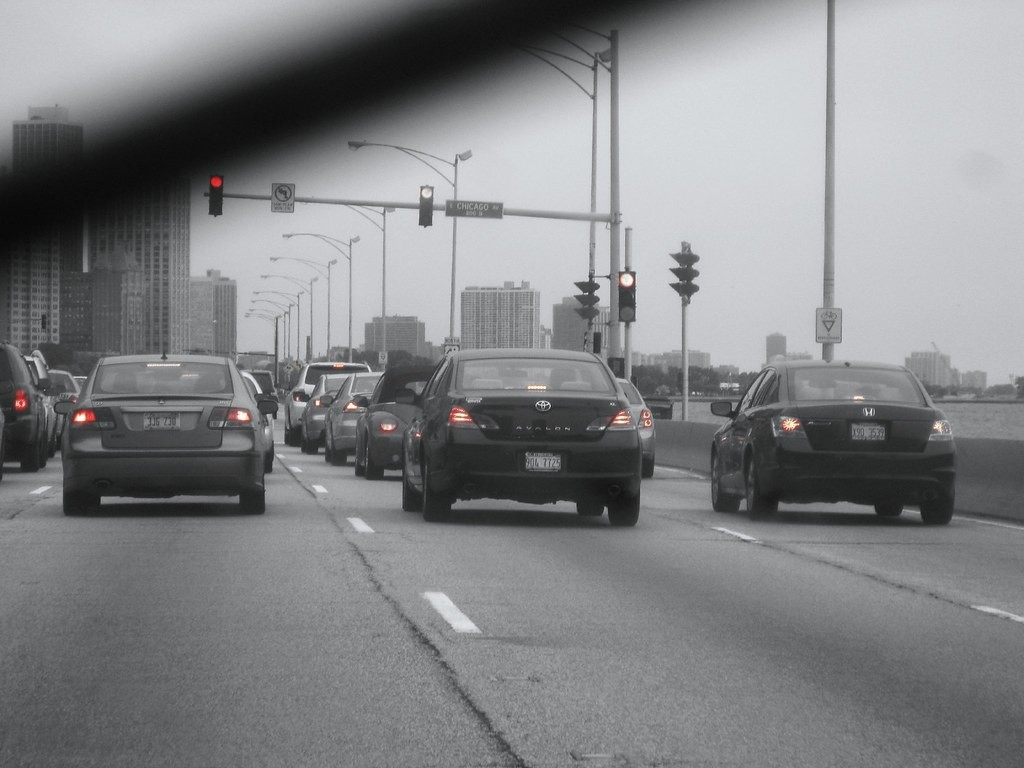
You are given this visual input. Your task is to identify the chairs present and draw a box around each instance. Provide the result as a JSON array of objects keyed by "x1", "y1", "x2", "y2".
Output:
[
  {"x1": 796, "y1": 382, "x2": 907, "y2": 402},
  {"x1": 112, "y1": 373, "x2": 224, "y2": 394},
  {"x1": 469, "y1": 368, "x2": 585, "y2": 393}
]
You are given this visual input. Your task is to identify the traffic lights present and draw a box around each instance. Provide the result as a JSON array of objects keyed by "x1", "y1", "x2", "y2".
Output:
[
  {"x1": 418, "y1": 185, "x2": 434, "y2": 225},
  {"x1": 208, "y1": 173, "x2": 224, "y2": 215},
  {"x1": 617, "y1": 271, "x2": 637, "y2": 323}
]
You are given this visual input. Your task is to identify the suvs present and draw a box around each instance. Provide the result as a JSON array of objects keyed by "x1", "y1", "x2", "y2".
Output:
[
  {"x1": 1, "y1": 340, "x2": 52, "y2": 473},
  {"x1": 279, "y1": 362, "x2": 372, "y2": 448}
]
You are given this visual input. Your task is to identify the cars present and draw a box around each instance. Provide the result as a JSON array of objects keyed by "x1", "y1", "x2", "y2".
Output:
[
  {"x1": 53, "y1": 354, "x2": 279, "y2": 515},
  {"x1": 293, "y1": 373, "x2": 353, "y2": 456},
  {"x1": 710, "y1": 358, "x2": 958, "y2": 526},
  {"x1": 353, "y1": 363, "x2": 436, "y2": 481},
  {"x1": 613, "y1": 377, "x2": 656, "y2": 479},
  {"x1": 24, "y1": 348, "x2": 285, "y2": 474},
  {"x1": 642, "y1": 394, "x2": 674, "y2": 421},
  {"x1": 395, "y1": 345, "x2": 644, "y2": 526},
  {"x1": 319, "y1": 371, "x2": 384, "y2": 466}
]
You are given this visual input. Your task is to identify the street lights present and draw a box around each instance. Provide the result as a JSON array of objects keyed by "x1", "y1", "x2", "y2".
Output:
[
  {"x1": 254, "y1": 290, "x2": 306, "y2": 361},
  {"x1": 270, "y1": 256, "x2": 336, "y2": 362},
  {"x1": 349, "y1": 140, "x2": 472, "y2": 351},
  {"x1": 283, "y1": 232, "x2": 360, "y2": 363},
  {"x1": 262, "y1": 273, "x2": 319, "y2": 362},
  {"x1": 251, "y1": 299, "x2": 295, "y2": 360}
]
